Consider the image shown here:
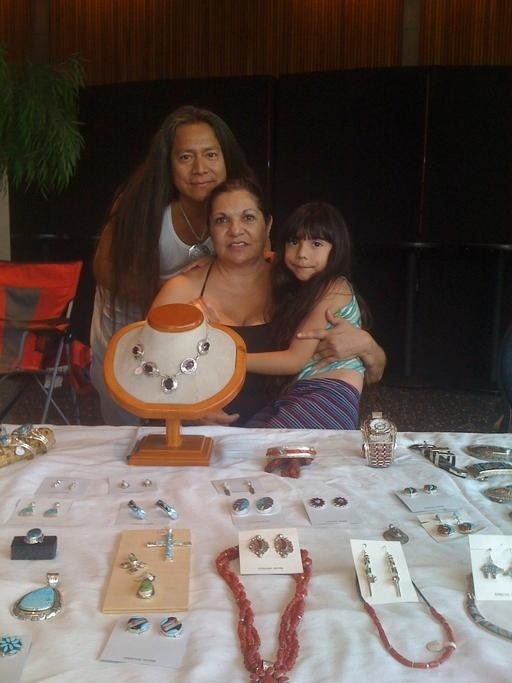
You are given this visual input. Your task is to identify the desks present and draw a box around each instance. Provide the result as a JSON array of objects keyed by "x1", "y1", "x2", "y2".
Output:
[{"x1": 1, "y1": 418, "x2": 512, "y2": 683}]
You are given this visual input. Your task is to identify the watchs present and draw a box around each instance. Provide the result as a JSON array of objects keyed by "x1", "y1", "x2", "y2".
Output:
[{"x1": 360, "y1": 410, "x2": 398, "y2": 469}]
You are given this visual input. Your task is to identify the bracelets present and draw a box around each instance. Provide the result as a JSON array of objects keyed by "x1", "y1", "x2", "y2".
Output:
[
  {"x1": 12, "y1": 422, "x2": 51, "y2": 453},
  {"x1": 0, "y1": 434, "x2": 36, "y2": 463},
  {"x1": 265, "y1": 444, "x2": 319, "y2": 461}
]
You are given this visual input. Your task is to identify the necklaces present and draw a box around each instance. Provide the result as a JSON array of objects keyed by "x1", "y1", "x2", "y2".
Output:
[
  {"x1": 216, "y1": 543, "x2": 312, "y2": 683},
  {"x1": 174, "y1": 197, "x2": 212, "y2": 261},
  {"x1": 466, "y1": 572, "x2": 512, "y2": 646},
  {"x1": 132, "y1": 326, "x2": 210, "y2": 393},
  {"x1": 356, "y1": 572, "x2": 457, "y2": 669}
]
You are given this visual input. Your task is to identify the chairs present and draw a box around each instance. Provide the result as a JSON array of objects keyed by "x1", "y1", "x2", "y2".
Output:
[{"x1": 0, "y1": 256, "x2": 86, "y2": 425}]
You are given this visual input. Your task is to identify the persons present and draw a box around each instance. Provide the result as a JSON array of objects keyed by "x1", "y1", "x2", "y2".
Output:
[
  {"x1": 144, "y1": 176, "x2": 387, "y2": 427},
  {"x1": 239, "y1": 200, "x2": 367, "y2": 429},
  {"x1": 89, "y1": 103, "x2": 256, "y2": 427}
]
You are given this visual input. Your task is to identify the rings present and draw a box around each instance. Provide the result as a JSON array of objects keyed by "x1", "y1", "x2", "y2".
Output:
[{"x1": 23, "y1": 527, "x2": 47, "y2": 549}]
